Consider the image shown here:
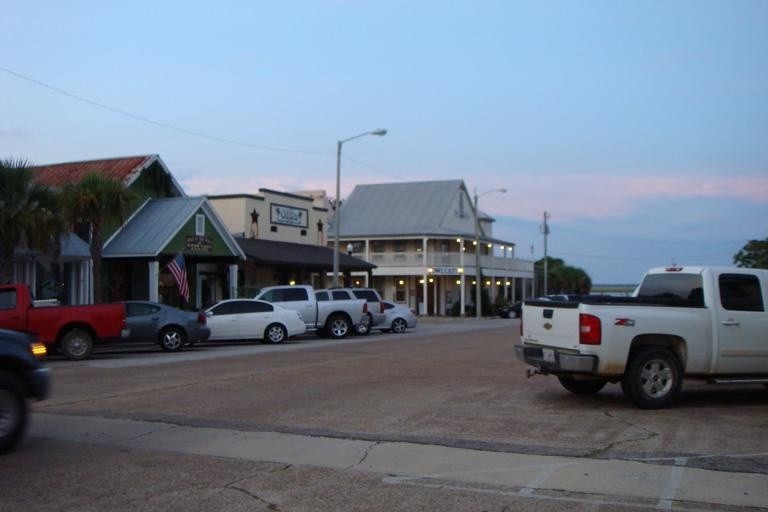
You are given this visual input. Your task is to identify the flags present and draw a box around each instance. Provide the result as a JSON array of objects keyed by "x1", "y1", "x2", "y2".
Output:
[{"x1": 166, "y1": 249, "x2": 189, "y2": 303}]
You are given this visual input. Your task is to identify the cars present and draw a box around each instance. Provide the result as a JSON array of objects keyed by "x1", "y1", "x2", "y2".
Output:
[
  {"x1": 370, "y1": 300, "x2": 418, "y2": 333},
  {"x1": 496, "y1": 301, "x2": 522, "y2": 319},
  {"x1": 183, "y1": 298, "x2": 306, "y2": 346},
  {"x1": 116, "y1": 301, "x2": 210, "y2": 351}
]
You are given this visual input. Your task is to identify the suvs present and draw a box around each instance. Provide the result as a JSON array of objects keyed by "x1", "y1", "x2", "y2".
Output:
[{"x1": 315, "y1": 286, "x2": 385, "y2": 335}]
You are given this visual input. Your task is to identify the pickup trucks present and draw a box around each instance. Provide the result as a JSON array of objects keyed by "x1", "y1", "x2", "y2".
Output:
[
  {"x1": 0, "y1": 283, "x2": 130, "y2": 360},
  {"x1": 513, "y1": 267, "x2": 767, "y2": 410},
  {"x1": 251, "y1": 285, "x2": 367, "y2": 339}
]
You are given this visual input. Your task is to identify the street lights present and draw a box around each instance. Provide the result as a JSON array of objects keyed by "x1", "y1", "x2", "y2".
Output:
[
  {"x1": 333, "y1": 129, "x2": 387, "y2": 289},
  {"x1": 473, "y1": 188, "x2": 506, "y2": 318}
]
